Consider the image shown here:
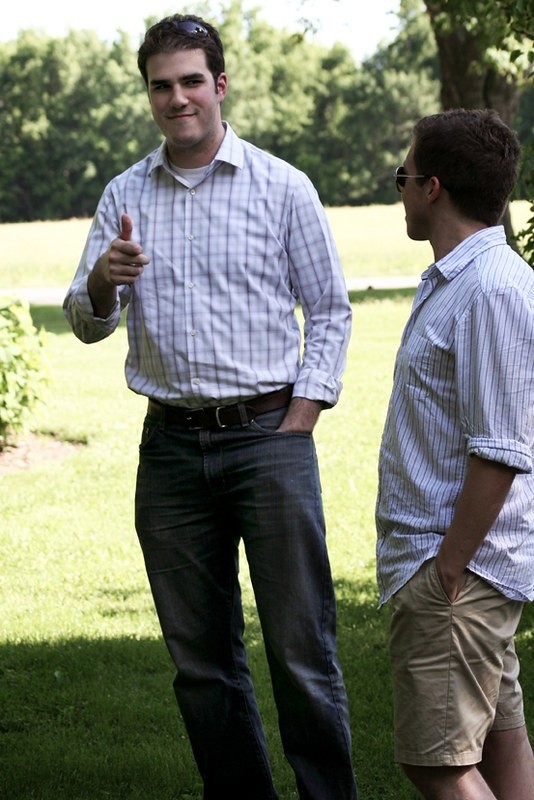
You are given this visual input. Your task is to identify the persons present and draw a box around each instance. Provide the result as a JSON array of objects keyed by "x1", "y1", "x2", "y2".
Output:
[
  {"x1": 372, "y1": 107, "x2": 534, "y2": 800},
  {"x1": 61, "y1": 13, "x2": 359, "y2": 800}
]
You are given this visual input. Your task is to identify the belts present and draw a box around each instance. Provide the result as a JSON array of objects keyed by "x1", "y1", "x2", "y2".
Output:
[{"x1": 147, "y1": 384, "x2": 295, "y2": 428}]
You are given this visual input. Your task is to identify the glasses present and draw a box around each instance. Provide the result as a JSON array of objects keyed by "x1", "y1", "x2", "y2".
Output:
[
  {"x1": 394, "y1": 166, "x2": 431, "y2": 192},
  {"x1": 145, "y1": 20, "x2": 209, "y2": 38}
]
